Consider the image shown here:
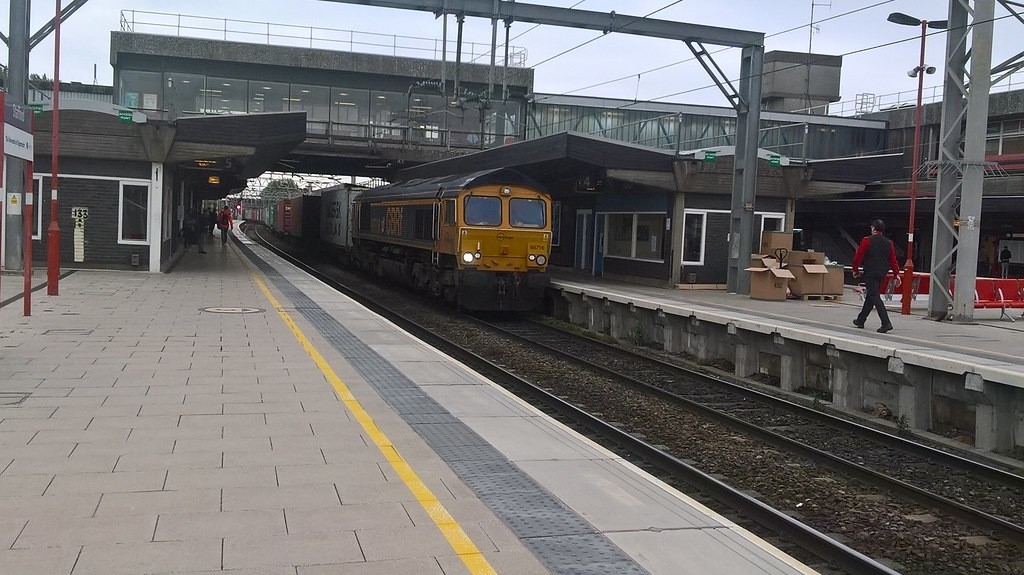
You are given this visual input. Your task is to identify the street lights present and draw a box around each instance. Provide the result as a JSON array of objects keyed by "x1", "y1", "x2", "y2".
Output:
[{"x1": 888, "y1": 12, "x2": 948, "y2": 315}]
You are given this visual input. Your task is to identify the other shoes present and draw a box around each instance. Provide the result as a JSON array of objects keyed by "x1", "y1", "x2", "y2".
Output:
[
  {"x1": 222, "y1": 243, "x2": 228, "y2": 246},
  {"x1": 198, "y1": 251, "x2": 207, "y2": 254}
]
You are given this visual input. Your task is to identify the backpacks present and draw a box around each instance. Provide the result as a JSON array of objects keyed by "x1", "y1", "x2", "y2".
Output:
[{"x1": 221, "y1": 212, "x2": 231, "y2": 225}]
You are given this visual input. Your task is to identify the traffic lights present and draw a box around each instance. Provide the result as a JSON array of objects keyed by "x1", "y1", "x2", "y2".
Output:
[{"x1": 237, "y1": 204, "x2": 241, "y2": 210}]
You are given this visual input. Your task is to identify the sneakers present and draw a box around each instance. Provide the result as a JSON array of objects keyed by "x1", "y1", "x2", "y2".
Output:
[
  {"x1": 852, "y1": 319, "x2": 865, "y2": 329},
  {"x1": 876, "y1": 323, "x2": 894, "y2": 333}
]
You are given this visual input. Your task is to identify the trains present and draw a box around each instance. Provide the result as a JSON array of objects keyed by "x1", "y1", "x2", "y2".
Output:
[{"x1": 246, "y1": 168, "x2": 557, "y2": 317}]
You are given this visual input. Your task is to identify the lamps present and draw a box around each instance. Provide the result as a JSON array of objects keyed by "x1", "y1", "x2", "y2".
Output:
[{"x1": 208, "y1": 171, "x2": 220, "y2": 183}]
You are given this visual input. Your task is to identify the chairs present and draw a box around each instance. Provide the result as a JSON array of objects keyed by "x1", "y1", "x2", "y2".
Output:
[{"x1": 853, "y1": 272, "x2": 1024, "y2": 322}]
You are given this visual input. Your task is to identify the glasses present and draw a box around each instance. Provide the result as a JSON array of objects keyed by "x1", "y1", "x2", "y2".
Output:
[{"x1": 870, "y1": 226, "x2": 874, "y2": 228}]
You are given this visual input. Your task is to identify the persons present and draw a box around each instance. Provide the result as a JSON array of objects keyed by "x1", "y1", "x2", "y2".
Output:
[
  {"x1": 853, "y1": 219, "x2": 902, "y2": 333},
  {"x1": 1000, "y1": 246, "x2": 1011, "y2": 279},
  {"x1": 183, "y1": 207, "x2": 217, "y2": 254},
  {"x1": 218, "y1": 206, "x2": 233, "y2": 245}
]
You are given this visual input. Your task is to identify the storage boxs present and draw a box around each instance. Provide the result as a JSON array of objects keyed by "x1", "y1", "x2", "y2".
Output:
[{"x1": 743, "y1": 230, "x2": 844, "y2": 301}]
